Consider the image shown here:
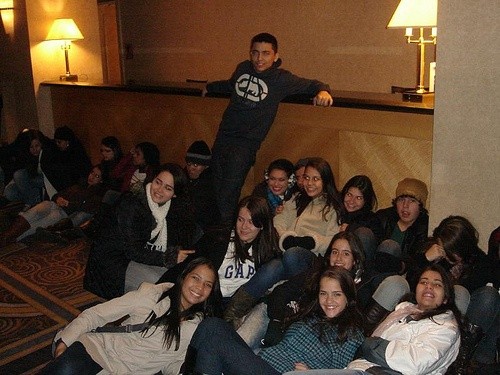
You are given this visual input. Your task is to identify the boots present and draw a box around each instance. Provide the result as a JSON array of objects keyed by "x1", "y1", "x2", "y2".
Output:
[
  {"x1": 288, "y1": 271, "x2": 307, "y2": 304},
  {"x1": 46, "y1": 217, "x2": 85, "y2": 243},
  {"x1": 356, "y1": 296, "x2": 394, "y2": 337},
  {"x1": 444, "y1": 320, "x2": 483, "y2": 375},
  {"x1": 222, "y1": 285, "x2": 257, "y2": 326},
  {"x1": 0, "y1": 214, "x2": 31, "y2": 246},
  {"x1": 36, "y1": 218, "x2": 74, "y2": 242}
]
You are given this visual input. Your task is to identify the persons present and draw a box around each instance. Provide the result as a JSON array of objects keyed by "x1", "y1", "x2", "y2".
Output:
[
  {"x1": 36, "y1": 253, "x2": 222, "y2": 375},
  {"x1": 0, "y1": 126, "x2": 500, "y2": 375},
  {"x1": 191, "y1": 32, "x2": 333, "y2": 268}
]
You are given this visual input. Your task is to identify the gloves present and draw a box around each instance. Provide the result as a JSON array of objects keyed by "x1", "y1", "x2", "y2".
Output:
[
  {"x1": 259, "y1": 320, "x2": 283, "y2": 348},
  {"x1": 282, "y1": 236, "x2": 315, "y2": 250},
  {"x1": 369, "y1": 251, "x2": 403, "y2": 274}
]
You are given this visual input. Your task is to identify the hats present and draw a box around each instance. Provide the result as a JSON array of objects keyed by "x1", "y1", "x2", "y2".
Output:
[
  {"x1": 184, "y1": 140, "x2": 211, "y2": 166},
  {"x1": 396, "y1": 178, "x2": 428, "y2": 207}
]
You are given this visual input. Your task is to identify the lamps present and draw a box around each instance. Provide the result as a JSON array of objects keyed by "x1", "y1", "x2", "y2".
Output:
[
  {"x1": 386, "y1": 0, "x2": 438, "y2": 103},
  {"x1": 46, "y1": 18, "x2": 84, "y2": 80}
]
